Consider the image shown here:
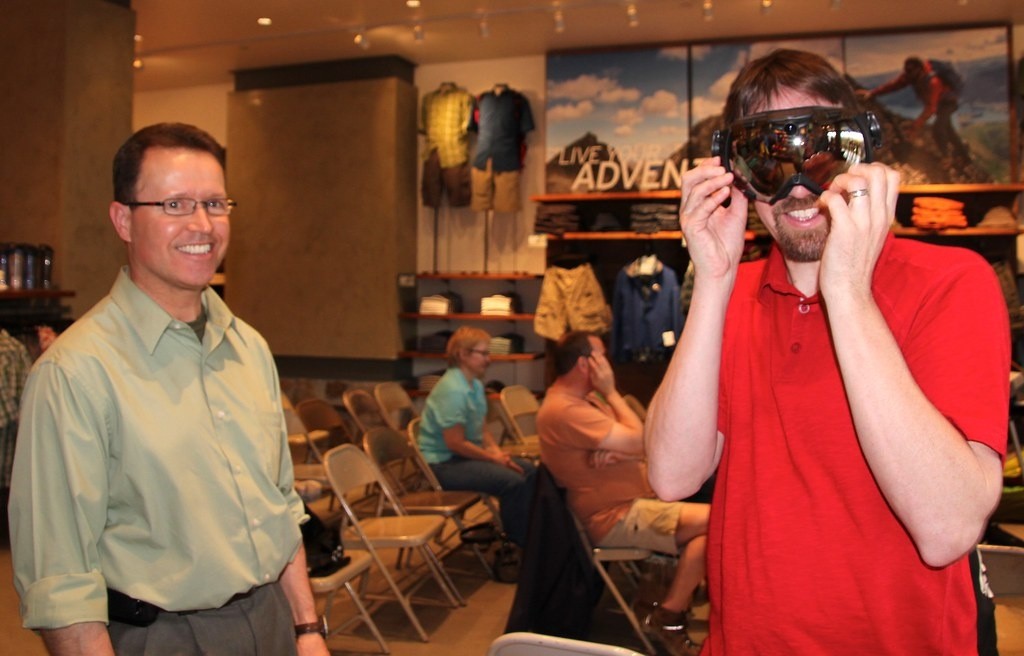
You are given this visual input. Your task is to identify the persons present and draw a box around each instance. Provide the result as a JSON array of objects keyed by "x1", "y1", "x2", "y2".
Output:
[
  {"x1": 641, "y1": 49, "x2": 1013, "y2": 656},
  {"x1": 0, "y1": 322, "x2": 32, "y2": 545},
  {"x1": 415, "y1": 81, "x2": 476, "y2": 210},
  {"x1": 463, "y1": 81, "x2": 535, "y2": 216},
  {"x1": 7, "y1": 123, "x2": 332, "y2": 655},
  {"x1": 854, "y1": 55, "x2": 975, "y2": 174},
  {"x1": 417, "y1": 325, "x2": 540, "y2": 583},
  {"x1": 534, "y1": 331, "x2": 717, "y2": 656}
]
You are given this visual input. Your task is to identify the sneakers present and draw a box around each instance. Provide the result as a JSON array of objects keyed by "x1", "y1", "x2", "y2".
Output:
[{"x1": 636, "y1": 603, "x2": 706, "y2": 656}]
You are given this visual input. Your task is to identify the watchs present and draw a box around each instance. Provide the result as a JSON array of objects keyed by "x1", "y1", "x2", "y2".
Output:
[{"x1": 293, "y1": 615, "x2": 329, "y2": 639}]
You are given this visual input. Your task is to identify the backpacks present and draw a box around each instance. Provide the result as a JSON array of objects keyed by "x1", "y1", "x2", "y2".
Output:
[{"x1": 920, "y1": 55, "x2": 966, "y2": 103}]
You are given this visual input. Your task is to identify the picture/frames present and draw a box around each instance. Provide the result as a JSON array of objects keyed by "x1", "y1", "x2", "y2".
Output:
[
  {"x1": 843, "y1": 23, "x2": 1015, "y2": 181},
  {"x1": 689, "y1": 34, "x2": 848, "y2": 158},
  {"x1": 541, "y1": 42, "x2": 692, "y2": 190}
]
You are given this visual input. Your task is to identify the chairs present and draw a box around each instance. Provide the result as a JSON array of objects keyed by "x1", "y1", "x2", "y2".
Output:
[{"x1": 268, "y1": 370, "x2": 1024, "y2": 656}]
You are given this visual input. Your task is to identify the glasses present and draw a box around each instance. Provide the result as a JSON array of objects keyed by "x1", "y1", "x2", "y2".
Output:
[
  {"x1": 122, "y1": 196, "x2": 238, "y2": 217},
  {"x1": 465, "y1": 347, "x2": 491, "y2": 359},
  {"x1": 709, "y1": 106, "x2": 883, "y2": 206}
]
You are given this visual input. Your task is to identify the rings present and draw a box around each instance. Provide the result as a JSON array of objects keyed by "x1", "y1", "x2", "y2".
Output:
[{"x1": 848, "y1": 188, "x2": 868, "y2": 201}]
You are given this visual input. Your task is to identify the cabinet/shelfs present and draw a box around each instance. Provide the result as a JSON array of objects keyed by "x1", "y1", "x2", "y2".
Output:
[
  {"x1": 0, "y1": 288, "x2": 80, "y2": 336},
  {"x1": 395, "y1": 269, "x2": 541, "y2": 365},
  {"x1": 530, "y1": 195, "x2": 1024, "y2": 334}
]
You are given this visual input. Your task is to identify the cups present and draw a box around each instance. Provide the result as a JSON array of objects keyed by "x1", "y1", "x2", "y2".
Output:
[{"x1": 0, "y1": 242, "x2": 52, "y2": 289}]
